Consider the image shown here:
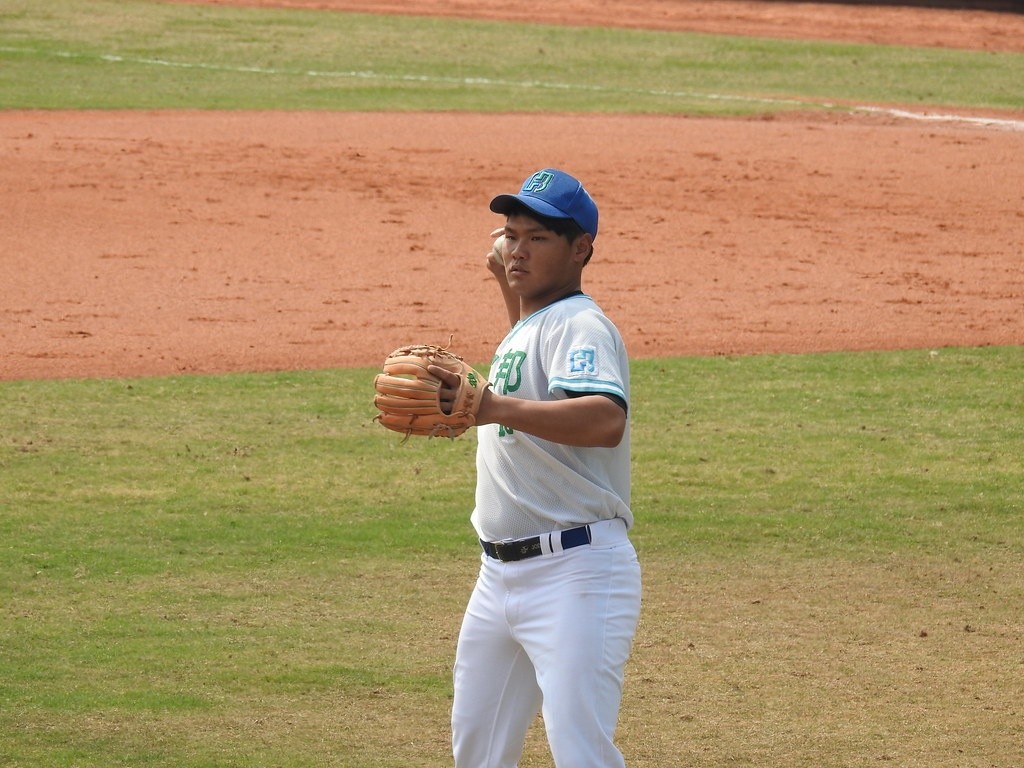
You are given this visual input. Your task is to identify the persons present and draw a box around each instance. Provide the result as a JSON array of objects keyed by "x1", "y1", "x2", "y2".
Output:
[{"x1": 373, "y1": 169, "x2": 644, "y2": 768}]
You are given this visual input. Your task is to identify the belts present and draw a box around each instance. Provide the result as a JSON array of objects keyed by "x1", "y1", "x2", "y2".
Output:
[{"x1": 478, "y1": 518, "x2": 592, "y2": 562}]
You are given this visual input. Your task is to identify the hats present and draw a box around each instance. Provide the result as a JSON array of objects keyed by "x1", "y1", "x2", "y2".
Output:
[{"x1": 489, "y1": 168, "x2": 598, "y2": 244}]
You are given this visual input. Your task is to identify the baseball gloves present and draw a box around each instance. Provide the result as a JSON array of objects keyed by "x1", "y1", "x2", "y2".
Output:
[{"x1": 372, "y1": 333, "x2": 493, "y2": 443}]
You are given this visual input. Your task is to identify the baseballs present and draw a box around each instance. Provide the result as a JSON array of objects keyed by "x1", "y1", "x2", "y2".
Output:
[{"x1": 492, "y1": 234, "x2": 505, "y2": 266}]
